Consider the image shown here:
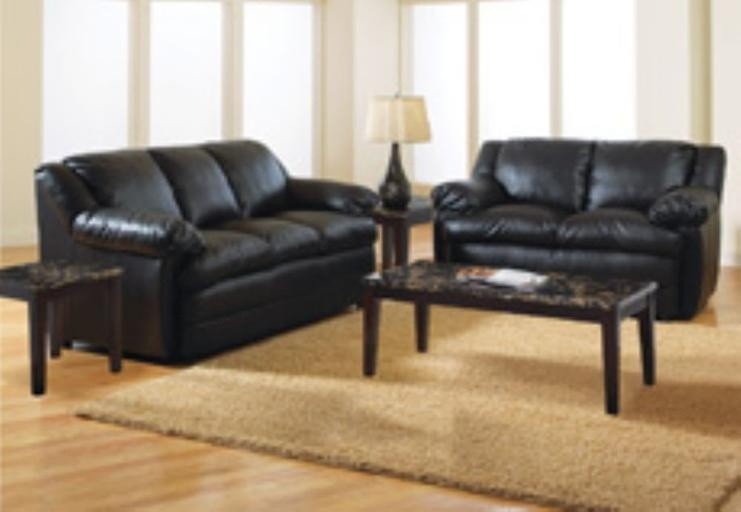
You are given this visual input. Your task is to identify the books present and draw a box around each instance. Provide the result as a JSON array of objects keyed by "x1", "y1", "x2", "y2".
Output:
[{"x1": 457, "y1": 264, "x2": 549, "y2": 291}]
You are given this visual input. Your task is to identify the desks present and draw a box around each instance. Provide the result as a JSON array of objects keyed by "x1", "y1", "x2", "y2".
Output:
[
  {"x1": 0, "y1": 260, "x2": 125, "y2": 394},
  {"x1": 371, "y1": 196, "x2": 433, "y2": 269}
]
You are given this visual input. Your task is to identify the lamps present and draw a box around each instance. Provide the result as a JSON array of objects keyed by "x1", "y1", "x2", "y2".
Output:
[{"x1": 366, "y1": 92, "x2": 430, "y2": 211}]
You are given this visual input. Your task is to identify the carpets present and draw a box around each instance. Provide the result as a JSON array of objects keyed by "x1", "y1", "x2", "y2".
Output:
[{"x1": 74, "y1": 299, "x2": 740, "y2": 512}]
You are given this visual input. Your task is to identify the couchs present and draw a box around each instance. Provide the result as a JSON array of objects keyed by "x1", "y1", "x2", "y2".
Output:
[
  {"x1": 33, "y1": 137, "x2": 380, "y2": 368},
  {"x1": 430, "y1": 138, "x2": 726, "y2": 322}
]
